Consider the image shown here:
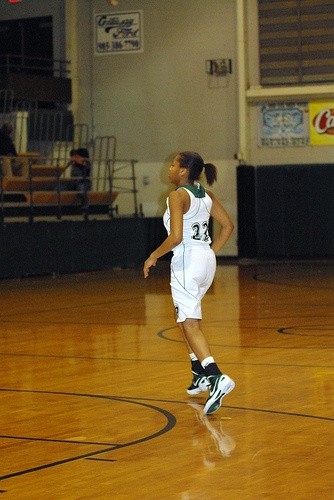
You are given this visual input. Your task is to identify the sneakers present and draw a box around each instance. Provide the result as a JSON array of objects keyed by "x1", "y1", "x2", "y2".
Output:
[
  {"x1": 187, "y1": 370, "x2": 210, "y2": 395},
  {"x1": 202, "y1": 374, "x2": 236, "y2": 414}
]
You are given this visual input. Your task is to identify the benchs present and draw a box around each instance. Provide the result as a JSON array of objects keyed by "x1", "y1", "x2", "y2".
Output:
[{"x1": 0, "y1": 152, "x2": 118, "y2": 215}]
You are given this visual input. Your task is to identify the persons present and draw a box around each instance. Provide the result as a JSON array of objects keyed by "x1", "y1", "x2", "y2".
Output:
[
  {"x1": 0, "y1": 124, "x2": 17, "y2": 156},
  {"x1": 143, "y1": 152, "x2": 235, "y2": 414},
  {"x1": 181, "y1": 402, "x2": 236, "y2": 499},
  {"x1": 60, "y1": 149, "x2": 93, "y2": 217}
]
